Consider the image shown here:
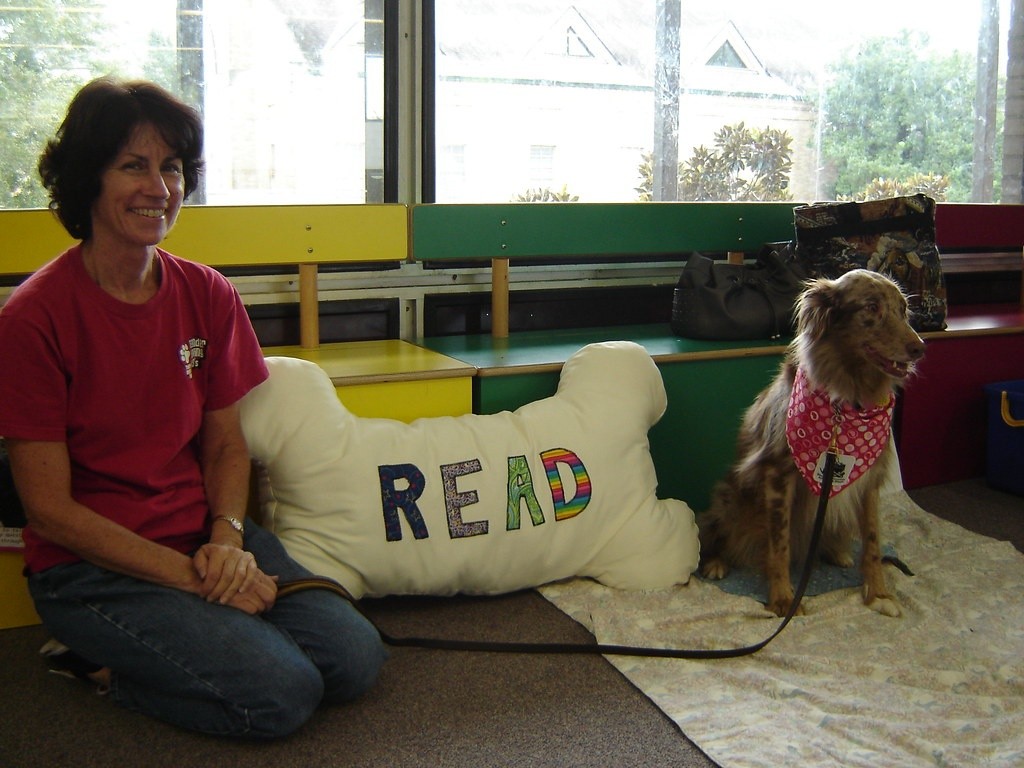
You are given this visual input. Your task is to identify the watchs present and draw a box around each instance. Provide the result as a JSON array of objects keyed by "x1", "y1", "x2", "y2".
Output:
[{"x1": 214, "y1": 514, "x2": 245, "y2": 535}]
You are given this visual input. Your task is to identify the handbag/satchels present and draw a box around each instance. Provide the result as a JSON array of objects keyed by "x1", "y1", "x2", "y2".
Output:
[
  {"x1": 980, "y1": 381, "x2": 1024, "y2": 487},
  {"x1": 792, "y1": 193, "x2": 949, "y2": 335},
  {"x1": 671, "y1": 240, "x2": 809, "y2": 341}
]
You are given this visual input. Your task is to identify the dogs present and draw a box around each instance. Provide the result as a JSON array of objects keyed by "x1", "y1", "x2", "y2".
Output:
[{"x1": 697, "y1": 267, "x2": 926, "y2": 619}]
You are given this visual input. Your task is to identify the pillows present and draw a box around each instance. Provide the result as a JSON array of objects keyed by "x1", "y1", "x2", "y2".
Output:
[{"x1": 216, "y1": 340, "x2": 698, "y2": 591}]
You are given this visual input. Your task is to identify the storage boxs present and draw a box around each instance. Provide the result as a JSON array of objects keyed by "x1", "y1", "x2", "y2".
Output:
[{"x1": 983, "y1": 379, "x2": 1024, "y2": 494}]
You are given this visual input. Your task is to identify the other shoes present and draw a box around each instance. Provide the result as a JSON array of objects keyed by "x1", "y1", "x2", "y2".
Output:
[{"x1": 36, "y1": 640, "x2": 112, "y2": 685}]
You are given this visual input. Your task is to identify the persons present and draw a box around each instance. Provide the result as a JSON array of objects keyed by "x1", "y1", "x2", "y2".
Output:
[{"x1": 0, "y1": 77, "x2": 386, "y2": 734}]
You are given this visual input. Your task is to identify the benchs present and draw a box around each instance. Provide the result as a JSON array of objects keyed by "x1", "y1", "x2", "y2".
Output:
[{"x1": 0, "y1": 204, "x2": 1024, "y2": 631}]
